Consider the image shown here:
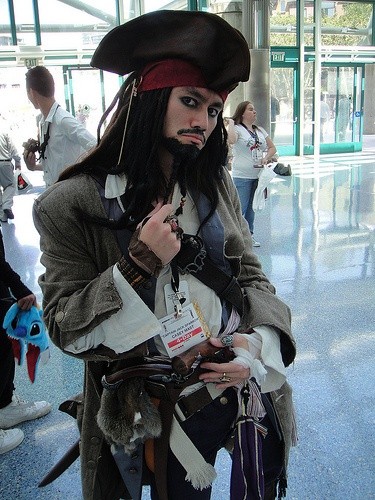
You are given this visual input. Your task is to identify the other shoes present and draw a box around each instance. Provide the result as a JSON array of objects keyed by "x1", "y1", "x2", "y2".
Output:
[
  {"x1": 3, "y1": 207, "x2": 14, "y2": 219},
  {"x1": 0, "y1": 214, "x2": 7, "y2": 222}
]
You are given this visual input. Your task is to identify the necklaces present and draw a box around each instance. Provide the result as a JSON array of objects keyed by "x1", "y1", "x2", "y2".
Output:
[{"x1": 170, "y1": 176, "x2": 187, "y2": 220}]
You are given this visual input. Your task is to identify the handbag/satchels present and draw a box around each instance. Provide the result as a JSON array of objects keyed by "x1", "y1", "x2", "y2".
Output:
[
  {"x1": 101, "y1": 309, "x2": 240, "y2": 459},
  {"x1": 14, "y1": 169, "x2": 33, "y2": 195},
  {"x1": 252, "y1": 164, "x2": 277, "y2": 213},
  {"x1": 264, "y1": 186, "x2": 268, "y2": 200},
  {"x1": 198, "y1": 378, "x2": 284, "y2": 500}
]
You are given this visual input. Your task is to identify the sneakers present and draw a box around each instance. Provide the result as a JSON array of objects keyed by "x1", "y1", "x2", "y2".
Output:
[
  {"x1": 250, "y1": 235, "x2": 261, "y2": 247},
  {"x1": 0, "y1": 427, "x2": 24, "y2": 454},
  {"x1": 0, "y1": 390, "x2": 52, "y2": 429}
]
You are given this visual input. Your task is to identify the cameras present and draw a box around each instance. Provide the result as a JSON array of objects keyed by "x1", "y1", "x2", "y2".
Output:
[{"x1": 23, "y1": 138, "x2": 40, "y2": 153}]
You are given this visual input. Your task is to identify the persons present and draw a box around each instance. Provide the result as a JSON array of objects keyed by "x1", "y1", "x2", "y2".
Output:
[
  {"x1": 317, "y1": 93, "x2": 353, "y2": 143},
  {"x1": 23, "y1": 66, "x2": 98, "y2": 189},
  {"x1": 32, "y1": 10, "x2": 296, "y2": 500},
  {"x1": 0, "y1": 113, "x2": 54, "y2": 458},
  {"x1": 271, "y1": 89, "x2": 280, "y2": 143},
  {"x1": 225, "y1": 101, "x2": 277, "y2": 247},
  {"x1": 75, "y1": 109, "x2": 86, "y2": 124}
]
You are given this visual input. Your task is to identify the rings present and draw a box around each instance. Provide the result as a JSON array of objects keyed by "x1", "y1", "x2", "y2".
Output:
[
  {"x1": 176, "y1": 228, "x2": 184, "y2": 239},
  {"x1": 221, "y1": 334, "x2": 234, "y2": 346},
  {"x1": 219, "y1": 372, "x2": 231, "y2": 384},
  {"x1": 168, "y1": 219, "x2": 178, "y2": 231}
]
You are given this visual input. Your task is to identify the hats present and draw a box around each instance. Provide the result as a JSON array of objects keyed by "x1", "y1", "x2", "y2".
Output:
[
  {"x1": 3, "y1": 302, "x2": 50, "y2": 384},
  {"x1": 90, "y1": 10, "x2": 250, "y2": 104}
]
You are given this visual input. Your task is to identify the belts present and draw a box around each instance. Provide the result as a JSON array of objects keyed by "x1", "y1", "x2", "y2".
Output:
[{"x1": 0, "y1": 160, "x2": 11, "y2": 161}]
